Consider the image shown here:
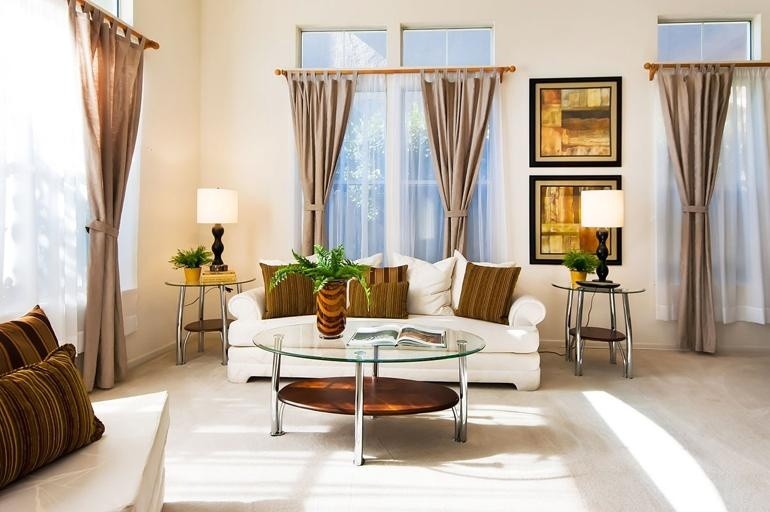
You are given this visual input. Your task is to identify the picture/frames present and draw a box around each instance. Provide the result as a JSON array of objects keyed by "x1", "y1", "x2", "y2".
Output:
[
  {"x1": 528, "y1": 175, "x2": 623, "y2": 266},
  {"x1": 528, "y1": 76, "x2": 621, "y2": 168}
]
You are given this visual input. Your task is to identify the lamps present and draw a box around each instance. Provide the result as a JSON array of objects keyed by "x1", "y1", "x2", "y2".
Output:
[
  {"x1": 196, "y1": 185, "x2": 239, "y2": 282},
  {"x1": 580, "y1": 189, "x2": 625, "y2": 287}
]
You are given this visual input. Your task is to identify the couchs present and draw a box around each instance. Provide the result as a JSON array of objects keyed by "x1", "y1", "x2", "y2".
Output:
[{"x1": 225, "y1": 261, "x2": 547, "y2": 392}]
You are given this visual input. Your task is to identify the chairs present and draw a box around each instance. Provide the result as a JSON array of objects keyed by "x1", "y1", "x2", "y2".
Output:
[{"x1": 0, "y1": 389, "x2": 171, "y2": 512}]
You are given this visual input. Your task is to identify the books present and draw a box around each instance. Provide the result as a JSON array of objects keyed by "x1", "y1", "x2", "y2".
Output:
[{"x1": 347, "y1": 324, "x2": 447, "y2": 348}]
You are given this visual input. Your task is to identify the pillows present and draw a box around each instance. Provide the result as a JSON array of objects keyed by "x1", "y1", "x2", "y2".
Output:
[
  {"x1": 344, "y1": 279, "x2": 409, "y2": 319},
  {"x1": 453, "y1": 250, "x2": 517, "y2": 312},
  {"x1": 0, "y1": 343, "x2": 106, "y2": 491},
  {"x1": 0, "y1": 305, "x2": 60, "y2": 376},
  {"x1": 259, "y1": 262, "x2": 315, "y2": 319},
  {"x1": 323, "y1": 253, "x2": 384, "y2": 269},
  {"x1": 344, "y1": 264, "x2": 410, "y2": 285},
  {"x1": 260, "y1": 253, "x2": 321, "y2": 266},
  {"x1": 453, "y1": 262, "x2": 521, "y2": 326},
  {"x1": 389, "y1": 248, "x2": 457, "y2": 318}
]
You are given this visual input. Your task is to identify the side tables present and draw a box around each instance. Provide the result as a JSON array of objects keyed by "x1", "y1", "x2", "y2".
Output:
[
  {"x1": 164, "y1": 275, "x2": 257, "y2": 365},
  {"x1": 551, "y1": 279, "x2": 645, "y2": 380}
]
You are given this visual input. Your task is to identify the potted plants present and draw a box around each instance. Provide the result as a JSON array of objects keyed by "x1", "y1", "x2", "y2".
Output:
[
  {"x1": 267, "y1": 243, "x2": 374, "y2": 339},
  {"x1": 561, "y1": 246, "x2": 603, "y2": 285},
  {"x1": 168, "y1": 240, "x2": 213, "y2": 283}
]
